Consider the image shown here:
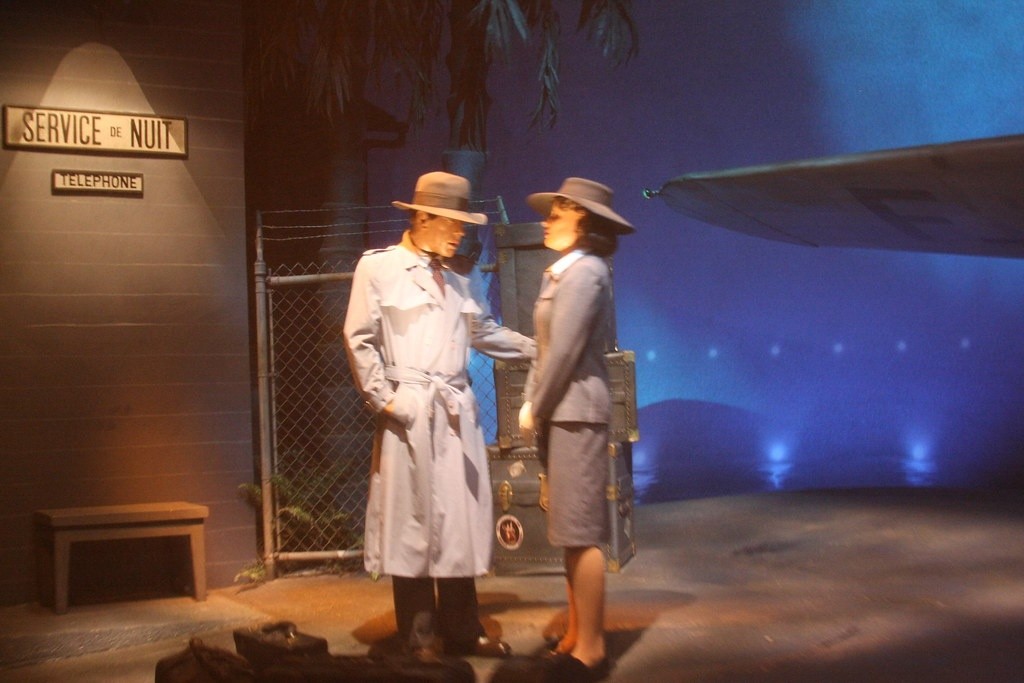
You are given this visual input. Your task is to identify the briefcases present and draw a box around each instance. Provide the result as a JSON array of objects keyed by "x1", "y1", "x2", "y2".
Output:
[{"x1": 233, "y1": 621, "x2": 328, "y2": 669}]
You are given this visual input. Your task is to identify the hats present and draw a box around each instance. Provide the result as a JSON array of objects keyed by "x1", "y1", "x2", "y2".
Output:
[
  {"x1": 527, "y1": 178, "x2": 636, "y2": 235},
  {"x1": 392, "y1": 171, "x2": 488, "y2": 225}
]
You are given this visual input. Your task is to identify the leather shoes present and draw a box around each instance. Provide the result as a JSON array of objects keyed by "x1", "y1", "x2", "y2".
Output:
[{"x1": 442, "y1": 633, "x2": 511, "y2": 656}]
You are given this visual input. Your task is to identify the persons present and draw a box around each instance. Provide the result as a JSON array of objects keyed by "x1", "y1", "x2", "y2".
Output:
[
  {"x1": 517, "y1": 177, "x2": 637, "y2": 683},
  {"x1": 342, "y1": 170, "x2": 536, "y2": 667}
]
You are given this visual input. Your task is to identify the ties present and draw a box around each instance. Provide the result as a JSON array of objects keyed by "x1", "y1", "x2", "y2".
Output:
[{"x1": 429, "y1": 258, "x2": 444, "y2": 299}]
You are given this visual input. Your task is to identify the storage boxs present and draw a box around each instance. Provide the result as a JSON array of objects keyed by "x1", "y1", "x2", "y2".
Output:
[
  {"x1": 497, "y1": 355, "x2": 642, "y2": 445},
  {"x1": 486, "y1": 448, "x2": 638, "y2": 572}
]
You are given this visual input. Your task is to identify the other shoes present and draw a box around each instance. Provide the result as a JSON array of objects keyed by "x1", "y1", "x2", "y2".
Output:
[{"x1": 533, "y1": 650, "x2": 609, "y2": 683}]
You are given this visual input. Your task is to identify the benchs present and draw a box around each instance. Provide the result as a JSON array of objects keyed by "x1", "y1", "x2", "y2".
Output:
[{"x1": 34, "y1": 501, "x2": 210, "y2": 616}]
